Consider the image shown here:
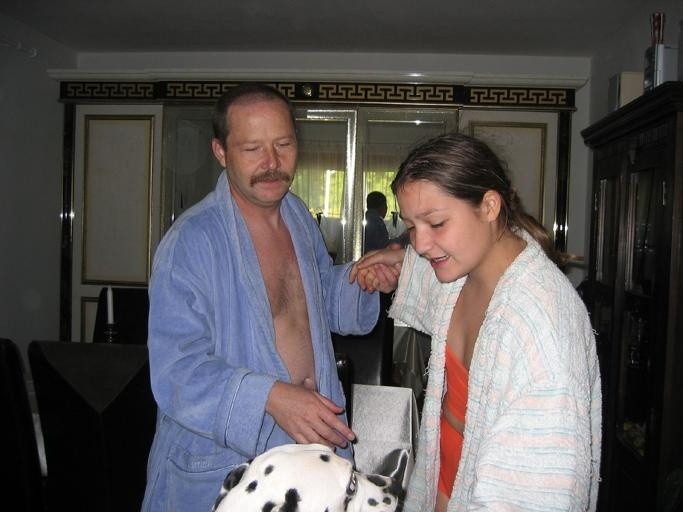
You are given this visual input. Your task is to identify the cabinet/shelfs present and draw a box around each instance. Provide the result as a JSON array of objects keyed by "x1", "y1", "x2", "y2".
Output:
[{"x1": 579, "y1": 80, "x2": 682, "y2": 511}]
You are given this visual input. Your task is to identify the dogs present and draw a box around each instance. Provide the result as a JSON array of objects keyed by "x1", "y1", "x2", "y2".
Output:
[{"x1": 209, "y1": 440, "x2": 405, "y2": 512}]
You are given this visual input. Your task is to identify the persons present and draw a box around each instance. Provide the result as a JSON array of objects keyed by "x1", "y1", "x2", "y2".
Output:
[
  {"x1": 364, "y1": 190, "x2": 402, "y2": 380},
  {"x1": 138, "y1": 81, "x2": 403, "y2": 511},
  {"x1": 344, "y1": 129, "x2": 603, "y2": 511}
]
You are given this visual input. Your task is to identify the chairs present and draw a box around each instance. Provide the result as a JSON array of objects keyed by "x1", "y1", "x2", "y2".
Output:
[
  {"x1": 0, "y1": 284, "x2": 159, "y2": 512},
  {"x1": 335, "y1": 351, "x2": 352, "y2": 409}
]
